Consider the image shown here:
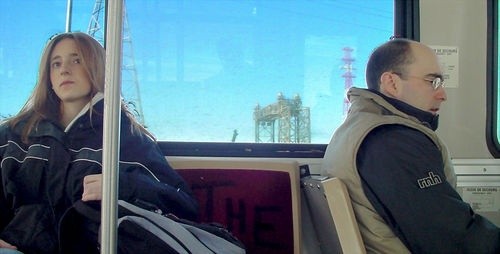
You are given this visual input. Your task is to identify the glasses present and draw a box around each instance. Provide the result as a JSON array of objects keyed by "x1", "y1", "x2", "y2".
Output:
[{"x1": 391, "y1": 72, "x2": 446, "y2": 89}]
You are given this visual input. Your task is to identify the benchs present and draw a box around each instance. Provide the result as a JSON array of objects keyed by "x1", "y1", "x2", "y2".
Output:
[{"x1": 165, "y1": 156, "x2": 301, "y2": 254}]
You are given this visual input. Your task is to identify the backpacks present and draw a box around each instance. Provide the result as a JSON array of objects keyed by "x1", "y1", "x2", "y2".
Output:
[{"x1": 117, "y1": 200, "x2": 246, "y2": 254}]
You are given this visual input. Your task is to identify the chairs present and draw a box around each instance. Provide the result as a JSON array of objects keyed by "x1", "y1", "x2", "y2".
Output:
[{"x1": 301, "y1": 175, "x2": 367, "y2": 254}]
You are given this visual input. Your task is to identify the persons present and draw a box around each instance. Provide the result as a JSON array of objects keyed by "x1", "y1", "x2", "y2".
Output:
[
  {"x1": 322, "y1": 38, "x2": 500, "y2": 254},
  {"x1": 0, "y1": 32, "x2": 246, "y2": 254}
]
